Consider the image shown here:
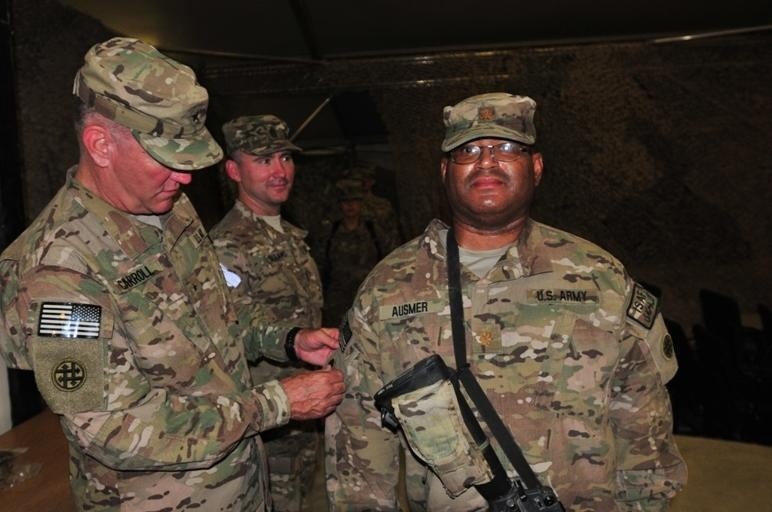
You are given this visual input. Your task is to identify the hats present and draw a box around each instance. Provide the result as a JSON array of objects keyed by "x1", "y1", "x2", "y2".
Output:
[
  {"x1": 336, "y1": 160, "x2": 374, "y2": 203},
  {"x1": 219, "y1": 113, "x2": 305, "y2": 159},
  {"x1": 72, "y1": 36, "x2": 226, "y2": 173},
  {"x1": 437, "y1": 91, "x2": 539, "y2": 155}
]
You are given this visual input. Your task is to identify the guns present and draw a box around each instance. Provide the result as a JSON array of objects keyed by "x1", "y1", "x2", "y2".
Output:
[{"x1": 375, "y1": 355, "x2": 566, "y2": 511}]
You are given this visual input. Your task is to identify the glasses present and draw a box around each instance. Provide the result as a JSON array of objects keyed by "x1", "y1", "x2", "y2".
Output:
[{"x1": 444, "y1": 141, "x2": 529, "y2": 164}]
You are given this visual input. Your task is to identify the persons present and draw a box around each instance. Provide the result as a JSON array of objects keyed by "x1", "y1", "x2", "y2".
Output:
[
  {"x1": 351, "y1": 160, "x2": 397, "y2": 224},
  {"x1": 0, "y1": 36, "x2": 345, "y2": 512},
  {"x1": 324, "y1": 91, "x2": 689, "y2": 512},
  {"x1": 305, "y1": 179, "x2": 399, "y2": 322},
  {"x1": 207, "y1": 113, "x2": 325, "y2": 512}
]
